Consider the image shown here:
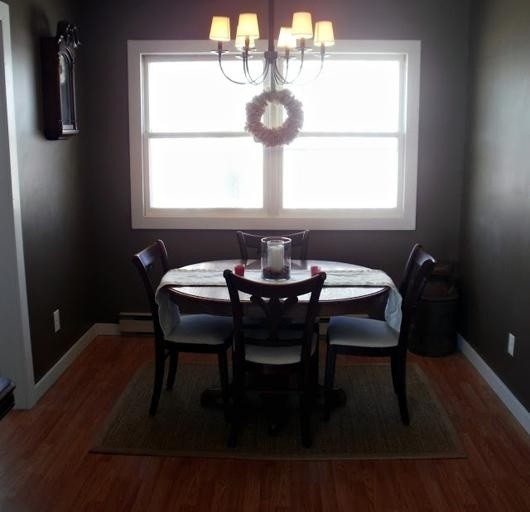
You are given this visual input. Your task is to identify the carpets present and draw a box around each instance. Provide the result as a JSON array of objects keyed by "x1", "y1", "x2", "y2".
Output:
[{"x1": 88, "y1": 360, "x2": 468, "y2": 462}]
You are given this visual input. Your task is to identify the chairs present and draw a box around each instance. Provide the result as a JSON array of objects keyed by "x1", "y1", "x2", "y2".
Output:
[
  {"x1": 131, "y1": 238, "x2": 237, "y2": 418},
  {"x1": 325, "y1": 243, "x2": 436, "y2": 425},
  {"x1": 235, "y1": 230, "x2": 311, "y2": 259},
  {"x1": 222, "y1": 268, "x2": 326, "y2": 451}
]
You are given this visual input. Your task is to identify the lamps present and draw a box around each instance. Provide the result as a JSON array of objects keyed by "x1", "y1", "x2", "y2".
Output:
[{"x1": 209, "y1": 0, "x2": 335, "y2": 87}]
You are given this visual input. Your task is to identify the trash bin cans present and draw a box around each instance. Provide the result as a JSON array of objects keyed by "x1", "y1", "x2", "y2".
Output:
[{"x1": 408, "y1": 262, "x2": 461, "y2": 357}]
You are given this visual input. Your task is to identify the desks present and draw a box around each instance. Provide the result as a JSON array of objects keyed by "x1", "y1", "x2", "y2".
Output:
[{"x1": 155, "y1": 259, "x2": 398, "y2": 408}]
